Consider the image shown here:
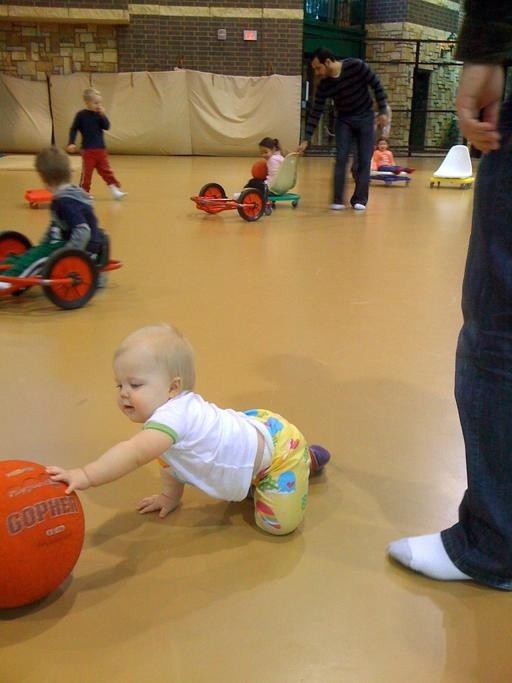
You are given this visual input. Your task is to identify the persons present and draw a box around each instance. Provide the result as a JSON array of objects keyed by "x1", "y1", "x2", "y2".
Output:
[
  {"x1": 46, "y1": 323, "x2": 332, "y2": 535},
  {"x1": 352, "y1": 93, "x2": 391, "y2": 184},
  {"x1": 68, "y1": 89, "x2": 130, "y2": 196},
  {"x1": 373, "y1": 138, "x2": 416, "y2": 176},
  {"x1": 386, "y1": 0, "x2": 512, "y2": 592},
  {"x1": 0, "y1": 148, "x2": 110, "y2": 296},
  {"x1": 234, "y1": 136, "x2": 285, "y2": 200},
  {"x1": 293, "y1": 47, "x2": 390, "y2": 211}
]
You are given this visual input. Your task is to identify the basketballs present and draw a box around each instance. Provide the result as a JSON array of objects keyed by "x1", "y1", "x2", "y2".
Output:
[
  {"x1": 1, "y1": 460, "x2": 85, "y2": 609},
  {"x1": 251, "y1": 159, "x2": 268, "y2": 181}
]
